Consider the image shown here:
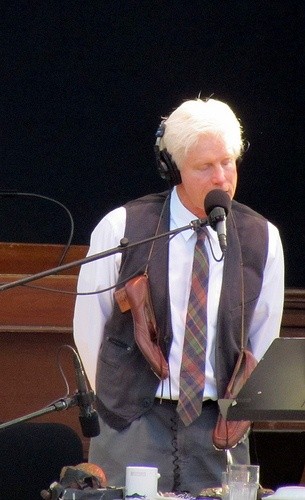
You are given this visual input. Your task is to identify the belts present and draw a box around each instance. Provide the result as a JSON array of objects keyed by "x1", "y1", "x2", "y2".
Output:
[{"x1": 154, "y1": 397, "x2": 213, "y2": 409}]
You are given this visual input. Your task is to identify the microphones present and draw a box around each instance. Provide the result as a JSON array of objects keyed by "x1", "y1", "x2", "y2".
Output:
[
  {"x1": 73, "y1": 353, "x2": 101, "y2": 438},
  {"x1": 203, "y1": 189, "x2": 231, "y2": 254}
]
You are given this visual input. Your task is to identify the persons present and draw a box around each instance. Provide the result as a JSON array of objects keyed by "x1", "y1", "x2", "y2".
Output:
[{"x1": 73, "y1": 96, "x2": 284, "y2": 498}]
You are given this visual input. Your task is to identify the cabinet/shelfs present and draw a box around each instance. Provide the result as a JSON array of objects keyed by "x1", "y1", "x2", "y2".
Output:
[{"x1": 0, "y1": 243, "x2": 94, "y2": 459}]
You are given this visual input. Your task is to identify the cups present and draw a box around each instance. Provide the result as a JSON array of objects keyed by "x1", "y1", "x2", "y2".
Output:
[
  {"x1": 221, "y1": 472, "x2": 249, "y2": 500},
  {"x1": 125, "y1": 466, "x2": 160, "y2": 500},
  {"x1": 229, "y1": 465, "x2": 259, "y2": 500}
]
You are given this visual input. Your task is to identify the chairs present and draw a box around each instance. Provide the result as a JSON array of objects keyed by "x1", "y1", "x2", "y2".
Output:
[{"x1": 0, "y1": 423, "x2": 83, "y2": 500}]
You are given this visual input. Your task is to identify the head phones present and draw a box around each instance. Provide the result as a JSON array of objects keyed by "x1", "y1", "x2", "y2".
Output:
[{"x1": 154, "y1": 117, "x2": 250, "y2": 185}]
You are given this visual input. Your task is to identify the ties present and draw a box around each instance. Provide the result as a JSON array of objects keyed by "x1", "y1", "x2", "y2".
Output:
[{"x1": 176, "y1": 220, "x2": 210, "y2": 427}]
